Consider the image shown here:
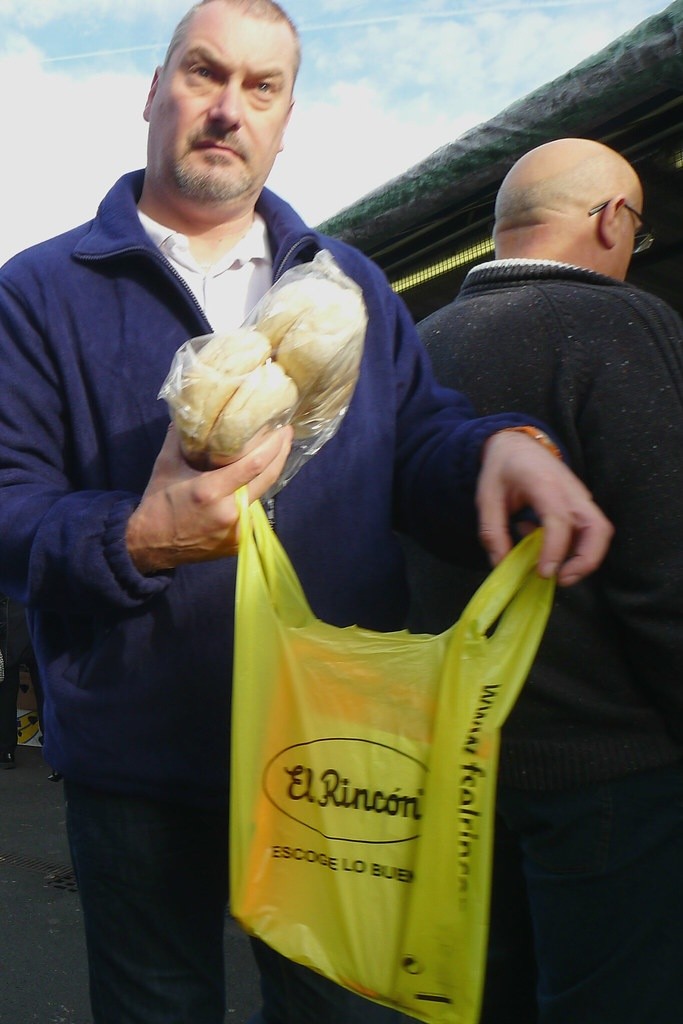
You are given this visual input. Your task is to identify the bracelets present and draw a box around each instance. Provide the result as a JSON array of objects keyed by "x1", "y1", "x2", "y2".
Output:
[{"x1": 508, "y1": 426, "x2": 562, "y2": 459}]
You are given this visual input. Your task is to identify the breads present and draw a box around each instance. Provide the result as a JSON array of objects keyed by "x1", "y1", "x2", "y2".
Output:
[{"x1": 172, "y1": 278, "x2": 369, "y2": 470}]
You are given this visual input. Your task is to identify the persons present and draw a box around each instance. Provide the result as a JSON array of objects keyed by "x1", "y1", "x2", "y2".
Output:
[
  {"x1": 0, "y1": 596, "x2": 44, "y2": 769},
  {"x1": 0, "y1": 0, "x2": 613, "y2": 1024},
  {"x1": 387, "y1": 138, "x2": 683, "y2": 1024}
]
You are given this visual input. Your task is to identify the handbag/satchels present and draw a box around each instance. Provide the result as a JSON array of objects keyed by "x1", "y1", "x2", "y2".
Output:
[{"x1": 228, "y1": 489, "x2": 557, "y2": 1024}]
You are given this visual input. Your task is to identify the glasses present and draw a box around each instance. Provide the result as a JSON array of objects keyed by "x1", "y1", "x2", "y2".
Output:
[{"x1": 587, "y1": 199, "x2": 657, "y2": 253}]
